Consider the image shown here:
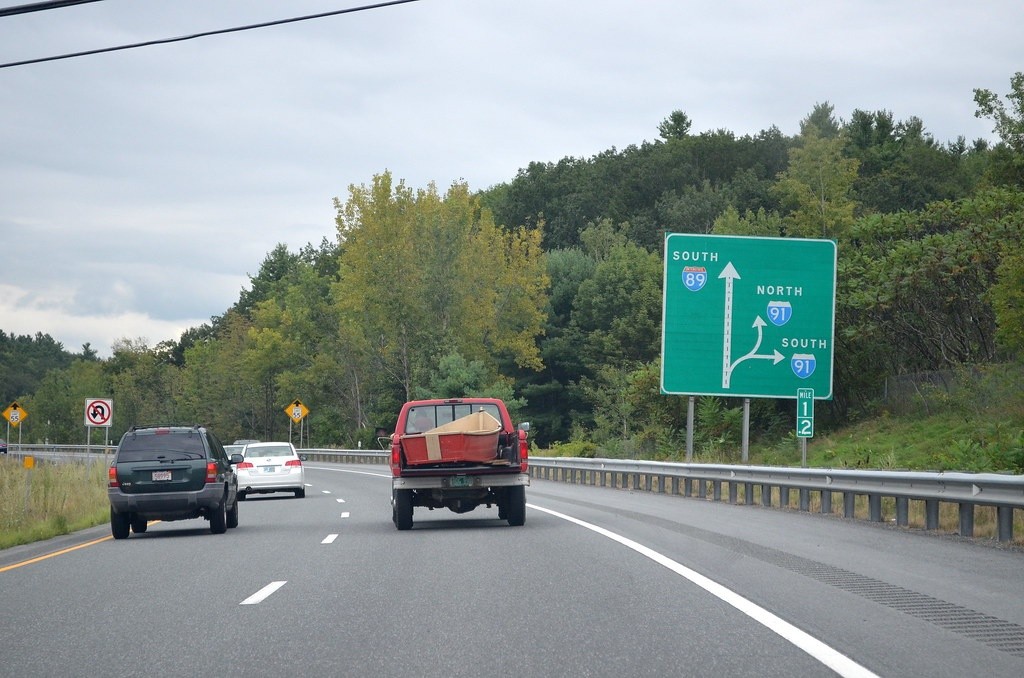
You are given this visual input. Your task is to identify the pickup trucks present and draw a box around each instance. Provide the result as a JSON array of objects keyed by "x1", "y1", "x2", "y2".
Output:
[{"x1": 378, "y1": 398, "x2": 530, "y2": 531}]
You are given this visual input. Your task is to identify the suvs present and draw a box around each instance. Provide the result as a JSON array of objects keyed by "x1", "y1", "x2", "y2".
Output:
[{"x1": 106, "y1": 423, "x2": 240, "y2": 540}]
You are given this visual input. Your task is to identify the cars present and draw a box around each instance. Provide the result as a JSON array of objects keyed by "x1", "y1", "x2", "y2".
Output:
[
  {"x1": 222, "y1": 443, "x2": 306, "y2": 500},
  {"x1": 0, "y1": 438, "x2": 7, "y2": 453}
]
